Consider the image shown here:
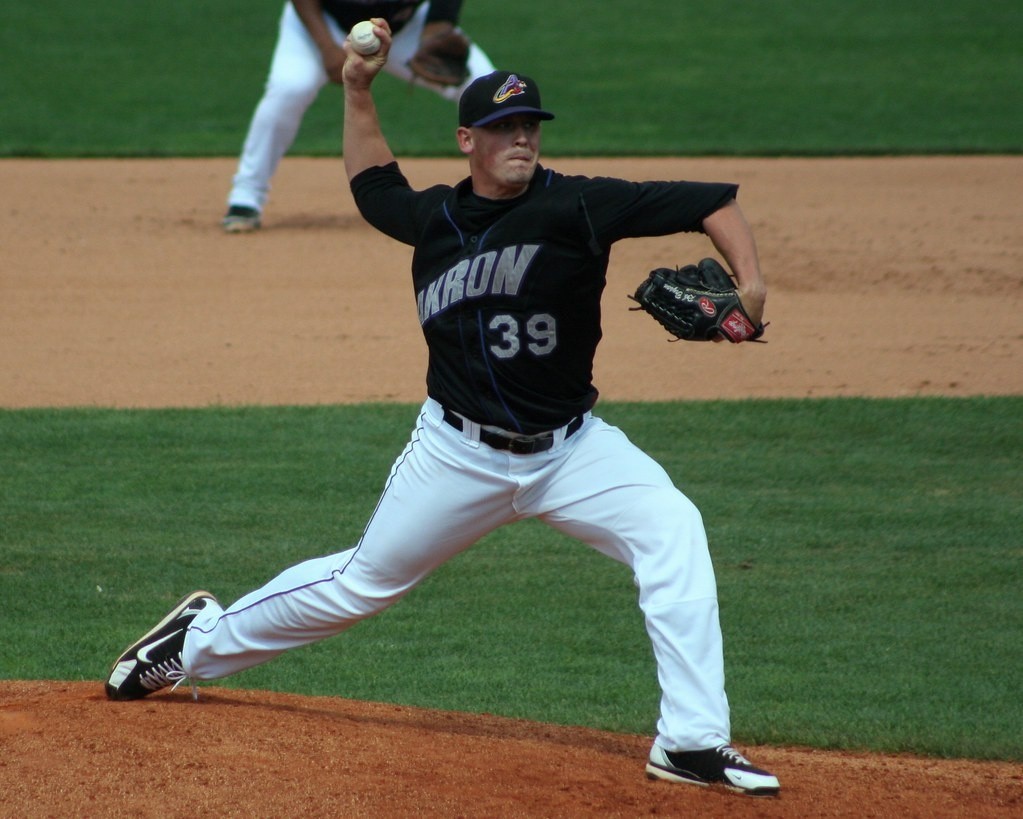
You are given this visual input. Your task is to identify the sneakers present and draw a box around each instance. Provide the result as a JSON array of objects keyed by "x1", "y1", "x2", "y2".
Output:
[
  {"x1": 104, "y1": 590, "x2": 218, "y2": 701},
  {"x1": 646, "y1": 743, "x2": 780, "y2": 799}
]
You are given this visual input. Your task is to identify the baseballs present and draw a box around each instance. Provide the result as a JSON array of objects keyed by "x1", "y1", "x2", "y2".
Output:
[{"x1": 349, "y1": 20, "x2": 383, "y2": 55}]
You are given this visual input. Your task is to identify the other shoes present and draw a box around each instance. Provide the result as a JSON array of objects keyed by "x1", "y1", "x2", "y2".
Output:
[{"x1": 222, "y1": 207, "x2": 260, "y2": 232}]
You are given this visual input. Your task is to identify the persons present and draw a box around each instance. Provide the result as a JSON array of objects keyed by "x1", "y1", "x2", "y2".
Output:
[
  {"x1": 106, "y1": 19, "x2": 781, "y2": 797},
  {"x1": 224, "y1": 0, "x2": 500, "y2": 233}
]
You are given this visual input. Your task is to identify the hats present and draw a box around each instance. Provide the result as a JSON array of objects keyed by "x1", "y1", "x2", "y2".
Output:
[{"x1": 459, "y1": 71, "x2": 555, "y2": 129}]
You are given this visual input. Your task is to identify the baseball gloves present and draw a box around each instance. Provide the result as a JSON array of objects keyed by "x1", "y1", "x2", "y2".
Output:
[
  {"x1": 633, "y1": 257, "x2": 766, "y2": 346},
  {"x1": 406, "y1": 30, "x2": 470, "y2": 87}
]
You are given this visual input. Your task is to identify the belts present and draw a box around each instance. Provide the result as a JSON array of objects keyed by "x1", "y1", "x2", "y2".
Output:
[{"x1": 442, "y1": 405, "x2": 584, "y2": 455}]
